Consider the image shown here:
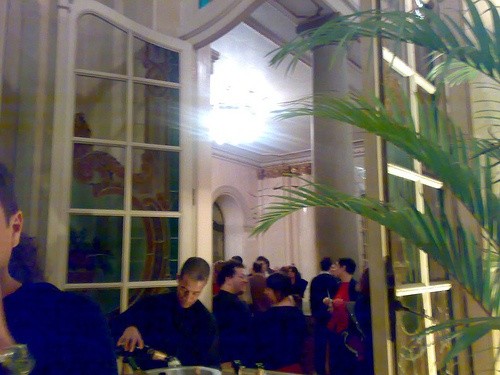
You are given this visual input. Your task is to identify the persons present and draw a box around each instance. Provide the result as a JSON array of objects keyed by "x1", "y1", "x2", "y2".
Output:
[
  {"x1": 0, "y1": 162, "x2": 117, "y2": 375},
  {"x1": 211, "y1": 259, "x2": 261, "y2": 371},
  {"x1": 310, "y1": 256, "x2": 342, "y2": 375},
  {"x1": 321, "y1": 257, "x2": 358, "y2": 375},
  {"x1": 213, "y1": 255, "x2": 410, "y2": 375},
  {"x1": 256, "y1": 272, "x2": 307, "y2": 375},
  {"x1": 112, "y1": 257, "x2": 221, "y2": 370}
]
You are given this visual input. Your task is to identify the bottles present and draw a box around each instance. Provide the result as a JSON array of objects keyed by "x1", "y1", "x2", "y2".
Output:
[{"x1": 116, "y1": 341, "x2": 177, "y2": 365}]
